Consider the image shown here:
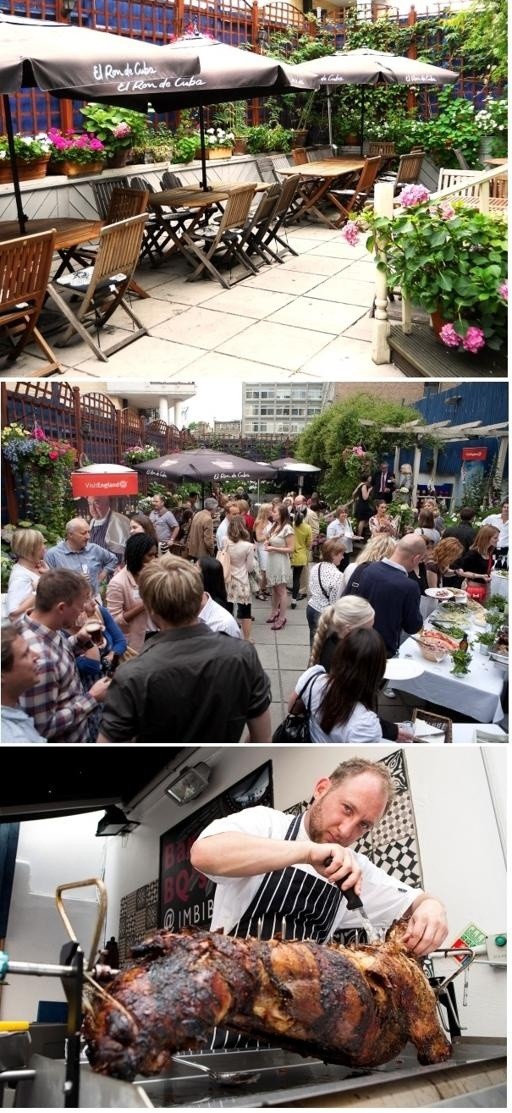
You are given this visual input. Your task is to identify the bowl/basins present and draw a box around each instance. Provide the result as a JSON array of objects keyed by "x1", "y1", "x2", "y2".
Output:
[{"x1": 416, "y1": 638, "x2": 452, "y2": 662}]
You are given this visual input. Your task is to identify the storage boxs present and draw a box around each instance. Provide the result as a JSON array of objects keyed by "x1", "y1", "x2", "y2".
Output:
[{"x1": 410, "y1": 707, "x2": 452, "y2": 743}]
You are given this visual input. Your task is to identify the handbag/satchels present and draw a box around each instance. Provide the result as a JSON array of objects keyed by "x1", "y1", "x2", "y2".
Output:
[
  {"x1": 249, "y1": 572, "x2": 260, "y2": 592},
  {"x1": 215, "y1": 544, "x2": 231, "y2": 583},
  {"x1": 272, "y1": 670, "x2": 324, "y2": 743},
  {"x1": 465, "y1": 554, "x2": 491, "y2": 602}
]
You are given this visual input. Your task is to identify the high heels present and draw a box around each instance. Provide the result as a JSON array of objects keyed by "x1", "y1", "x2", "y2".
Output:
[
  {"x1": 266, "y1": 611, "x2": 279, "y2": 622},
  {"x1": 271, "y1": 618, "x2": 286, "y2": 630},
  {"x1": 291, "y1": 603, "x2": 296, "y2": 609}
]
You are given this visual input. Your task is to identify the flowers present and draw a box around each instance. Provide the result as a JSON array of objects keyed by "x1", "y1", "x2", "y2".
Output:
[
  {"x1": 342, "y1": 183, "x2": 508, "y2": 366},
  {"x1": 47, "y1": 127, "x2": 109, "y2": 167},
  {"x1": 196, "y1": 127, "x2": 236, "y2": 148},
  {"x1": 0, "y1": 130, "x2": 53, "y2": 167},
  {"x1": 354, "y1": 115, "x2": 401, "y2": 142},
  {"x1": 473, "y1": 110, "x2": 505, "y2": 136},
  {"x1": 78, "y1": 104, "x2": 134, "y2": 153}
]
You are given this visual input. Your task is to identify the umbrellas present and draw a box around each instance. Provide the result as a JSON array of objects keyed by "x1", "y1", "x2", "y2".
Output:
[
  {"x1": 48, "y1": 33, "x2": 321, "y2": 268},
  {"x1": 131, "y1": 445, "x2": 278, "y2": 510},
  {"x1": 261, "y1": 458, "x2": 321, "y2": 477},
  {"x1": 0, "y1": 11, "x2": 200, "y2": 235},
  {"x1": 254, "y1": 461, "x2": 271, "y2": 503},
  {"x1": 292, "y1": 46, "x2": 460, "y2": 158}
]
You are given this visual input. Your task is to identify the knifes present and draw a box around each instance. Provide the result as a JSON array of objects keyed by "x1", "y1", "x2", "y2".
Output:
[{"x1": 325, "y1": 857, "x2": 380, "y2": 945}]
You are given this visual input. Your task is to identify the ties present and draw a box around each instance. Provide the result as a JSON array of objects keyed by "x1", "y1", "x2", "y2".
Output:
[{"x1": 382, "y1": 473, "x2": 385, "y2": 492}]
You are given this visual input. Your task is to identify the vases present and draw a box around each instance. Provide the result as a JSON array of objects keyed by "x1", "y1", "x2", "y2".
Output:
[
  {"x1": 430, "y1": 303, "x2": 461, "y2": 351},
  {"x1": 0, "y1": 155, "x2": 50, "y2": 184},
  {"x1": 107, "y1": 146, "x2": 133, "y2": 169},
  {"x1": 47, "y1": 161, "x2": 105, "y2": 179},
  {"x1": 477, "y1": 134, "x2": 494, "y2": 171},
  {"x1": 194, "y1": 146, "x2": 236, "y2": 160},
  {"x1": 362, "y1": 141, "x2": 395, "y2": 159}
]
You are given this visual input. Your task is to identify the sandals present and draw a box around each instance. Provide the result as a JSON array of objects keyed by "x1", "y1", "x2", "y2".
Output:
[
  {"x1": 256, "y1": 592, "x2": 268, "y2": 601},
  {"x1": 262, "y1": 588, "x2": 272, "y2": 596}
]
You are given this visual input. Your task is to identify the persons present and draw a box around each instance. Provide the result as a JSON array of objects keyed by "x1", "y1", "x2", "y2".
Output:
[
  {"x1": 173, "y1": 757, "x2": 448, "y2": 1055},
  {"x1": 353, "y1": 461, "x2": 398, "y2": 542}
]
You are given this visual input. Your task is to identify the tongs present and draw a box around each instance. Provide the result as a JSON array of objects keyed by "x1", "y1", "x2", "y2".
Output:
[{"x1": 428, "y1": 619, "x2": 455, "y2": 631}]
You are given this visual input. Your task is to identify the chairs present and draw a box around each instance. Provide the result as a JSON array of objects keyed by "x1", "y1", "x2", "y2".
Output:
[
  {"x1": 130, "y1": 177, "x2": 168, "y2": 257},
  {"x1": 237, "y1": 172, "x2": 302, "y2": 264},
  {"x1": 89, "y1": 175, "x2": 157, "y2": 265},
  {"x1": 454, "y1": 148, "x2": 470, "y2": 170},
  {"x1": 326, "y1": 154, "x2": 382, "y2": 230},
  {"x1": 46, "y1": 212, "x2": 151, "y2": 364},
  {"x1": 437, "y1": 166, "x2": 486, "y2": 197},
  {"x1": 185, "y1": 182, "x2": 258, "y2": 282},
  {"x1": 292, "y1": 147, "x2": 311, "y2": 165},
  {"x1": 0, "y1": 227, "x2": 65, "y2": 377},
  {"x1": 69, "y1": 187, "x2": 152, "y2": 299},
  {"x1": 230, "y1": 181, "x2": 284, "y2": 261},
  {"x1": 374, "y1": 150, "x2": 427, "y2": 196},
  {"x1": 255, "y1": 156, "x2": 280, "y2": 182},
  {"x1": 378, "y1": 149, "x2": 424, "y2": 177},
  {"x1": 159, "y1": 171, "x2": 190, "y2": 232}
]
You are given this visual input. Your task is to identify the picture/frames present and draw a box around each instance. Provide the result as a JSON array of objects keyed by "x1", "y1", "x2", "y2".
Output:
[
  {"x1": 154, "y1": 758, "x2": 275, "y2": 933},
  {"x1": 283, "y1": 748, "x2": 425, "y2": 894}
]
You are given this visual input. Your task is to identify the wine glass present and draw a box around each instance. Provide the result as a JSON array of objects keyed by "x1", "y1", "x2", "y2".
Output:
[
  {"x1": 402, "y1": 721, "x2": 415, "y2": 743},
  {"x1": 433, "y1": 652, "x2": 444, "y2": 668},
  {"x1": 265, "y1": 533, "x2": 270, "y2": 541}
]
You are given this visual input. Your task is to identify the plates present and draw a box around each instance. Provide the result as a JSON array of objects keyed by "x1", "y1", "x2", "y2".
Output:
[
  {"x1": 346, "y1": 535, "x2": 363, "y2": 540},
  {"x1": 425, "y1": 588, "x2": 453, "y2": 599},
  {"x1": 449, "y1": 588, "x2": 467, "y2": 598},
  {"x1": 490, "y1": 570, "x2": 508, "y2": 579},
  {"x1": 383, "y1": 658, "x2": 424, "y2": 681}
]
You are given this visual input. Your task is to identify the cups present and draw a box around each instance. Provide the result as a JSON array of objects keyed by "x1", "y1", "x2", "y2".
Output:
[
  {"x1": 85, "y1": 619, "x2": 104, "y2": 648},
  {"x1": 82, "y1": 564, "x2": 89, "y2": 576}
]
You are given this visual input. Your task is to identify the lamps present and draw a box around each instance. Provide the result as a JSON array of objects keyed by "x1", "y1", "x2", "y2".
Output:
[
  {"x1": 163, "y1": 760, "x2": 213, "y2": 809},
  {"x1": 95, "y1": 802, "x2": 142, "y2": 838}
]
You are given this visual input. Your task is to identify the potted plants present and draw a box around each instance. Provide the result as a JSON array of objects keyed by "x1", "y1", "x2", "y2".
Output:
[
  {"x1": 484, "y1": 593, "x2": 508, "y2": 614},
  {"x1": 219, "y1": 100, "x2": 251, "y2": 156},
  {"x1": 473, "y1": 631, "x2": 498, "y2": 657},
  {"x1": 337, "y1": 118, "x2": 362, "y2": 146},
  {"x1": 450, "y1": 649, "x2": 474, "y2": 679},
  {"x1": 484, "y1": 610, "x2": 506, "y2": 633},
  {"x1": 286, "y1": 89, "x2": 315, "y2": 148}
]
